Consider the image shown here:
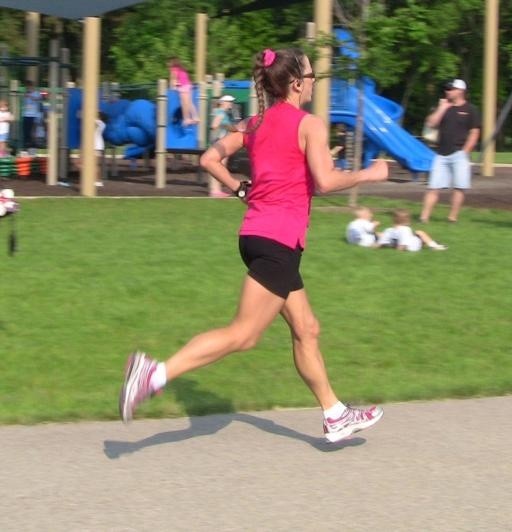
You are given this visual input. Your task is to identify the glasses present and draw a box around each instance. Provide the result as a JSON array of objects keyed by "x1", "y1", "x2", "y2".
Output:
[{"x1": 289, "y1": 73, "x2": 314, "y2": 83}]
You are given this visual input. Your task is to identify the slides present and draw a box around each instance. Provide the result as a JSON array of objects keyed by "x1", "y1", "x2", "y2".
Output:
[{"x1": 334, "y1": 87, "x2": 437, "y2": 172}]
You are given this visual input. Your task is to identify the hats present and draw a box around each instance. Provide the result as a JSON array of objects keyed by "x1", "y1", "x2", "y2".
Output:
[
  {"x1": 216, "y1": 95, "x2": 236, "y2": 101},
  {"x1": 442, "y1": 79, "x2": 467, "y2": 89}
]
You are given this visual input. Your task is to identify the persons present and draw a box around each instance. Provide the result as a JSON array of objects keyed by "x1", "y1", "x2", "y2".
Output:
[
  {"x1": 91, "y1": 112, "x2": 109, "y2": 187},
  {"x1": 343, "y1": 207, "x2": 381, "y2": 249},
  {"x1": 21, "y1": 80, "x2": 43, "y2": 148},
  {"x1": 117, "y1": 47, "x2": 386, "y2": 442},
  {"x1": 377, "y1": 208, "x2": 449, "y2": 253},
  {"x1": 165, "y1": 55, "x2": 200, "y2": 127},
  {"x1": 416, "y1": 78, "x2": 482, "y2": 223},
  {"x1": 206, "y1": 95, "x2": 237, "y2": 198}
]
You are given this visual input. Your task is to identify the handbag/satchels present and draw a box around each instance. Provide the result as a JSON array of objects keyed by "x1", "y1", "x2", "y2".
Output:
[{"x1": 422, "y1": 108, "x2": 439, "y2": 143}]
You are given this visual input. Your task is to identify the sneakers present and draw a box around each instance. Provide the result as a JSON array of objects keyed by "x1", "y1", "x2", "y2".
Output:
[
  {"x1": 322, "y1": 405, "x2": 384, "y2": 444},
  {"x1": 120, "y1": 349, "x2": 156, "y2": 422}
]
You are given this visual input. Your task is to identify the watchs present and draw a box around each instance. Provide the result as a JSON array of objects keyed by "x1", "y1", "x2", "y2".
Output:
[{"x1": 236, "y1": 181, "x2": 246, "y2": 198}]
